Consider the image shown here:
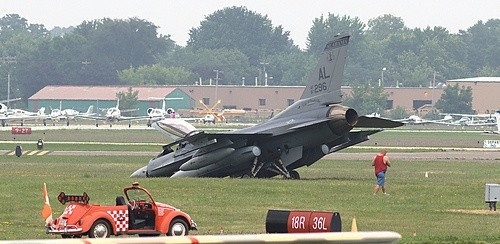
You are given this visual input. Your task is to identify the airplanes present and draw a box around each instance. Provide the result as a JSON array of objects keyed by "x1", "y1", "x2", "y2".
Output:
[
  {"x1": 129, "y1": 97, "x2": 207, "y2": 127},
  {"x1": 391, "y1": 114, "x2": 453, "y2": 126},
  {"x1": 439, "y1": 112, "x2": 500, "y2": 126},
  {"x1": 89, "y1": 97, "x2": 140, "y2": 128},
  {"x1": 129, "y1": 34, "x2": 405, "y2": 180},
  {"x1": 0, "y1": 97, "x2": 102, "y2": 127}
]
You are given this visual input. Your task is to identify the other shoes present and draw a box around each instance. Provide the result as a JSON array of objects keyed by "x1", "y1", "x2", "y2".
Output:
[{"x1": 383, "y1": 194, "x2": 390, "y2": 195}]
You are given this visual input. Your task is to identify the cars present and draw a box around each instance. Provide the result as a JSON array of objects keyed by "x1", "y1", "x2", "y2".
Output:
[
  {"x1": 41, "y1": 182, "x2": 198, "y2": 237},
  {"x1": 204, "y1": 114, "x2": 216, "y2": 125}
]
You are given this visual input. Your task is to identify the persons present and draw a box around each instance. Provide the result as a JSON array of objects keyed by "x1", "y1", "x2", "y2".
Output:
[
  {"x1": 146, "y1": 116, "x2": 152, "y2": 127},
  {"x1": 171, "y1": 111, "x2": 175, "y2": 118},
  {"x1": 372, "y1": 148, "x2": 391, "y2": 196}
]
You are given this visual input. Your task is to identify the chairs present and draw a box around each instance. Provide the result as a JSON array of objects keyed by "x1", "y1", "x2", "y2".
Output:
[{"x1": 115, "y1": 196, "x2": 145, "y2": 228}]
